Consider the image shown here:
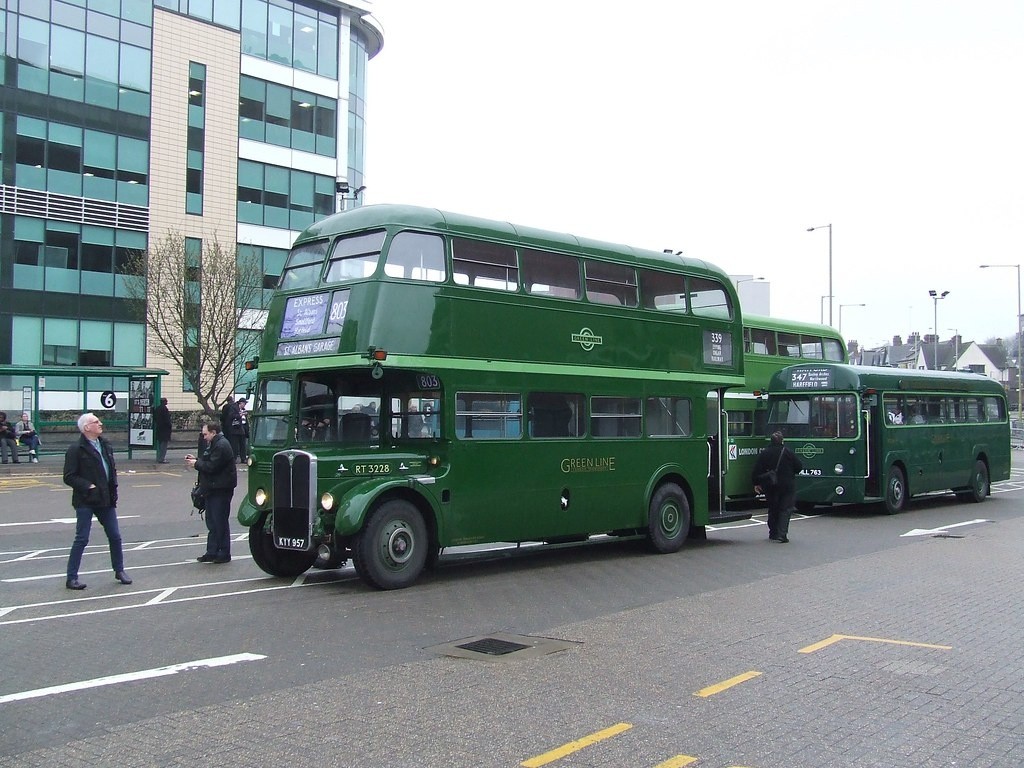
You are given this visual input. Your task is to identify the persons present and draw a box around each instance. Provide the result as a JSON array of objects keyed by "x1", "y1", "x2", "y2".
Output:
[
  {"x1": 516, "y1": 392, "x2": 573, "y2": 437},
  {"x1": 155, "y1": 398, "x2": 172, "y2": 463},
  {"x1": 220, "y1": 396, "x2": 250, "y2": 464},
  {"x1": 0, "y1": 412, "x2": 20, "y2": 464},
  {"x1": 888, "y1": 405, "x2": 924, "y2": 425},
  {"x1": 752, "y1": 432, "x2": 803, "y2": 543},
  {"x1": 350, "y1": 402, "x2": 380, "y2": 425},
  {"x1": 185, "y1": 423, "x2": 238, "y2": 562},
  {"x1": 409, "y1": 403, "x2": 433, "y2": 436},
  {"x1": 15, "y1": 412, "x2": 39, "y2": 463},
  {"x1": 62, "y1": 413, "x2": 132, "y2": 589}
]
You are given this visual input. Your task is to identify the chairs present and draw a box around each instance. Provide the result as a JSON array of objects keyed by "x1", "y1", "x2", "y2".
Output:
[
  {"x1": 910, "y1": 416, "x2": 1000, "y2": 424},
  {"x1": 338, "y1": 411, "x2": 371, "y2": 439}
]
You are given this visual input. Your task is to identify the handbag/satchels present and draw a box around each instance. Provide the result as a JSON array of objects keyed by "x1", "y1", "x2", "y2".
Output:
[
  {"x1": 190, "y1": 483, "x2": 206, "y2": 513},
  {"x1": 758, "y1": 470, "x2": 776, "y2": 489}
]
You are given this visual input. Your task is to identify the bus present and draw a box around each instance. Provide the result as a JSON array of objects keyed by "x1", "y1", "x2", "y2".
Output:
[
  {"x1": 236, "y1": 202, "x2": 751, "y2": 590},
  {"x1": 754, "y1": 362, "x2": 1012, "y2": 515},
  {"x1": 723, "y1": 316, "x2": 849, "y2": 499}
]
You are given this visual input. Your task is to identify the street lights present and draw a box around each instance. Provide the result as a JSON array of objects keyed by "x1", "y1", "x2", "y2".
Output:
[
  {"x1": 737, "y1": 278, "x2": 765, "y2": 295},
  {"x1": 821, "y1": 296, "x2": 834, "y2": 325},
  {"x1": 948, "y1": 329, "x2": 958, "y2": 371},
  {"x1": 336, "y1": 181, "x2": 366, "y2": 209},
  {"x1": 929, "y1": 290, "x2": 950, "y2": 371},
  {"x1": 912, "y1": 332, "x2": 919, "y2": 362},
  {"x1": 807, "y1": 224, "x2": 832, "y2": 325},
  {"x1": 980, "y1": 265, "x2": 1022, "y2": 421},
  {"x1": 839, "y1": 304, "x2": 866, "y2": 334}
]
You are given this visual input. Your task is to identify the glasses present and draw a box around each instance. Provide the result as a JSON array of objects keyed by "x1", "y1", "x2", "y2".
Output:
[{"x1": 87, "y1": 419, "x2": 101, "y2": 426}]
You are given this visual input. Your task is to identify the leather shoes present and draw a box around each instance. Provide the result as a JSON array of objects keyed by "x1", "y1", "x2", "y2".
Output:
[
  {"x1": 115, "y1": 570, "x2": 132, "y2": 584},
  {"x1": 66, "y1": 580, "x2": 85, "y2": 591}
]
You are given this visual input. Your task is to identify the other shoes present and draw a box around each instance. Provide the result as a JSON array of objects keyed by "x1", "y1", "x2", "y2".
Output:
[
  {"x1": 2, "y1": 460, "x2": 8, "y2": 464},
  {"x1": 241, "y1": 460, "x2": 246, "y2": 464},
  {"x1": 28, "y1": 449, "x2": 36, "y2": 455},
  {"x1": 32, "y1": 458, "x2": 38, "y2": 463},
  {"x1": 770, "y1": 533, "x2": 789, "y2": 543},
  {"x1": 214, "y1": 557, "x2": 230, "y2": 564},
  {"x1": 13, "y1": 460, "x2": 20, "y2": 463},
  {"x1": 197, "y1": 552, "x2": 215, "y2": 562},
  {"x1": 158, "y1": 459, "x2": 169, "y2": 463}
]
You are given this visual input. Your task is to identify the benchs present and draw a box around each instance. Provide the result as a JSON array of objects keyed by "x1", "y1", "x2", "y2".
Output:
[{"x1": 0, "y1": 442, "x2": 38, "y2": 462}]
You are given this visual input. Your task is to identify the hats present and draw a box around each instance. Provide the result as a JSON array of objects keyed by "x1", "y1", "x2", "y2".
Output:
[{"x1": 240, "y1": 397, "x2": 248, "y2": 403}]
